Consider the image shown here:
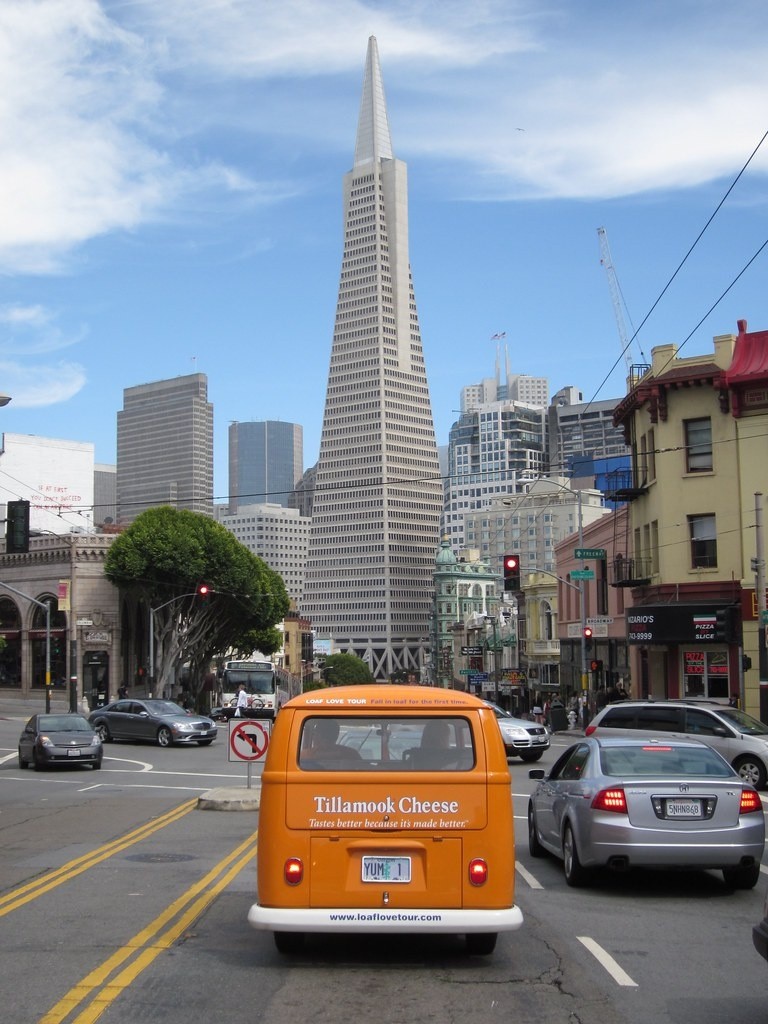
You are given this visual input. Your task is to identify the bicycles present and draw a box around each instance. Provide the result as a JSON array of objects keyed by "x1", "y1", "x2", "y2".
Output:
[{"x1": 229, "y1": 688, "x2": 263, "y2": 713}]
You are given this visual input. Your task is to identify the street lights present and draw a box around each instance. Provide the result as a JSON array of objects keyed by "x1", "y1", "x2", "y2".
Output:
[
  {"x1": 516, "y1": 478, "x2": 590, "y2": 731},
  {"x1": 29, "y1": 526, "x2": 78, "y2": 713}
]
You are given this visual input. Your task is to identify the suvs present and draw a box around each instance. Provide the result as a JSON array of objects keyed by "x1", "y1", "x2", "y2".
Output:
[
  {"x1": 583, "y1": 699, "x2": 768, "y2": 792},
  {"x1": 388, "y1": 699, "x2": 550, "y2": 764}
]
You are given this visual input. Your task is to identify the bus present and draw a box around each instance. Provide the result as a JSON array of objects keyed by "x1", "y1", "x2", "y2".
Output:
[{"x1": 218, "y1": 660, "x2": 302, "y2": 723}]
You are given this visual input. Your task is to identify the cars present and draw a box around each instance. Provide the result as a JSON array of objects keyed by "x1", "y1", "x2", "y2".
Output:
[
  {"x1": 338, "y1": 729, "x2": 422, "y2": 760},
  {"x1": 527, "y1": 734, "x2": 765, "y2": 891},
  {"x1": 87, "y1": 698, "x2": 218, "y2": 748},
  {"x1": 18, "y1": 714, "x2": 103, "y2": 771}
]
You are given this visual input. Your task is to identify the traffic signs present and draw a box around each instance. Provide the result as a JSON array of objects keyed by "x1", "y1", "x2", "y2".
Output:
[{"x1": 575, "y1": 548, "x2": 606, "y2": 560}]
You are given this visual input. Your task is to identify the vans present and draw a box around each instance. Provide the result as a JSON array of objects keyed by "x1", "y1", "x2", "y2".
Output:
[{"x1": 247, "y1": 685, "x2": 523, "y2": 959}]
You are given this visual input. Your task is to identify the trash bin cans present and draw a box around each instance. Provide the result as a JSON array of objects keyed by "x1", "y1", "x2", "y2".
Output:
[{"x1": 548, "y1": 699, "x2": 569, "y2": 732}]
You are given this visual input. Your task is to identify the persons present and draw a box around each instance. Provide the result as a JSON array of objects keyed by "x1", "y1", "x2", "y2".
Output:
[
  {"x1": 301, "y1": 720, "x2": 362, "y2": 760},
  {"x1": 729, "y1": 692, "x2": 739, "y2": 708},
  {"x1": 596, "y1": 684, "x2": 607, "y2": 712},
  {"x1": 420, "y1": 723, "x2": 451, "y2": 749},
  {"x1": 237, "y1": 685, "x2": 248, "y2": 718},
  {"x1": 118, "y1": 680, "x2": 129, "y2": 699},
  {"x1": 534, "y1": 697, "x2": 551, "y2": 725},
  {"x1": 609, "y1": 682, "x2": 627, "y2": 702},
  {"x1": 571, "y1": 690, "x2": 580, "y2": 723}
]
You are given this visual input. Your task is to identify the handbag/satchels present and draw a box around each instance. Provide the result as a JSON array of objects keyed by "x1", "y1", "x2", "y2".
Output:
[
  {"x1": 234, "y1": 708, "x2": 240, "y2": 716},
  {"x1": 124, "y1": 695, "x2": 128, "y2": 699},
  {"x1": 532, "y1": 706, "x2": 543, "y2": 713}
]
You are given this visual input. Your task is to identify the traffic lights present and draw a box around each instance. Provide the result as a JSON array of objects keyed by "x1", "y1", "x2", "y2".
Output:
[
  {"x1": 199, "y1": 586, "x2": 210, "y2": 609},
  {"x1": 138, "y1": 668, "x2": 147, "y2": 677},
  {"x1": 591, "y1": 660, "x2": 603, "y2": 672},
  {"x1": 504, "y1": 555, "x2": 520, "y2": 591},
  {"x1": 714, "y1": 606, "x2": 742, "y2": 646},
  {"x1": 584, "y1": 627, "x2": 592, "y2": 652}
]
